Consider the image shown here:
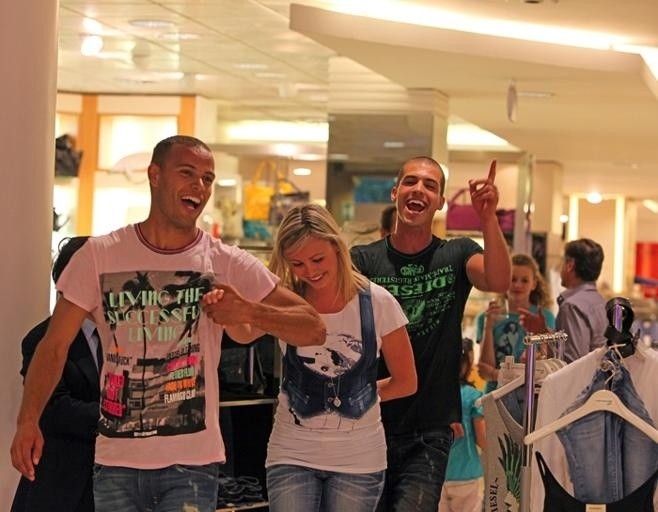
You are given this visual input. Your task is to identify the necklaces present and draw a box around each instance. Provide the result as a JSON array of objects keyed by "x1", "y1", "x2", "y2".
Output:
[{"x1": 327, "y1": 363, "x2": 346, "y2": 409}]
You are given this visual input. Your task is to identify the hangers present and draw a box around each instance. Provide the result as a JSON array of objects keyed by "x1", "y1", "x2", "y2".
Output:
[
  {"x1": 481, "y1": 331, "x2": 569, "y2": 404},
  {"x1": 520, "y1": 357, "x2": 657, "y2": 447}
]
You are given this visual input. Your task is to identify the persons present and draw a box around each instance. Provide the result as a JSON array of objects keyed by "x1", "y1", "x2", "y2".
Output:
[
  {"x1": 380, "y1": 205, "x2": 397, "y2": 241},
  {"x1": 547, "y1": 237, "x2": 611, "y2": 365},
  {"x1": 476, "y1": 254, "x2": 554, "y2": 423},
  {"x1": 202, "y1": 205, "x2": 417, "y2": 512},
  {"x1": 437, "y1": 337, "x2": 488, "y2": 511},
  {"x1": 347, "y1": 156, "x2": 513, "y2": 512},
  {"x1": 9, "y1": 135, "x2": 327, "y2": 512},
  {"x1": 8, "y1": 237, "x2": 103, "y2": 512}
]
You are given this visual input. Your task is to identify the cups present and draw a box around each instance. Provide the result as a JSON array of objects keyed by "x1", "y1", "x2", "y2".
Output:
[{"x1": 491, "y1": 296, "x2": 510, "y2": 321}]
